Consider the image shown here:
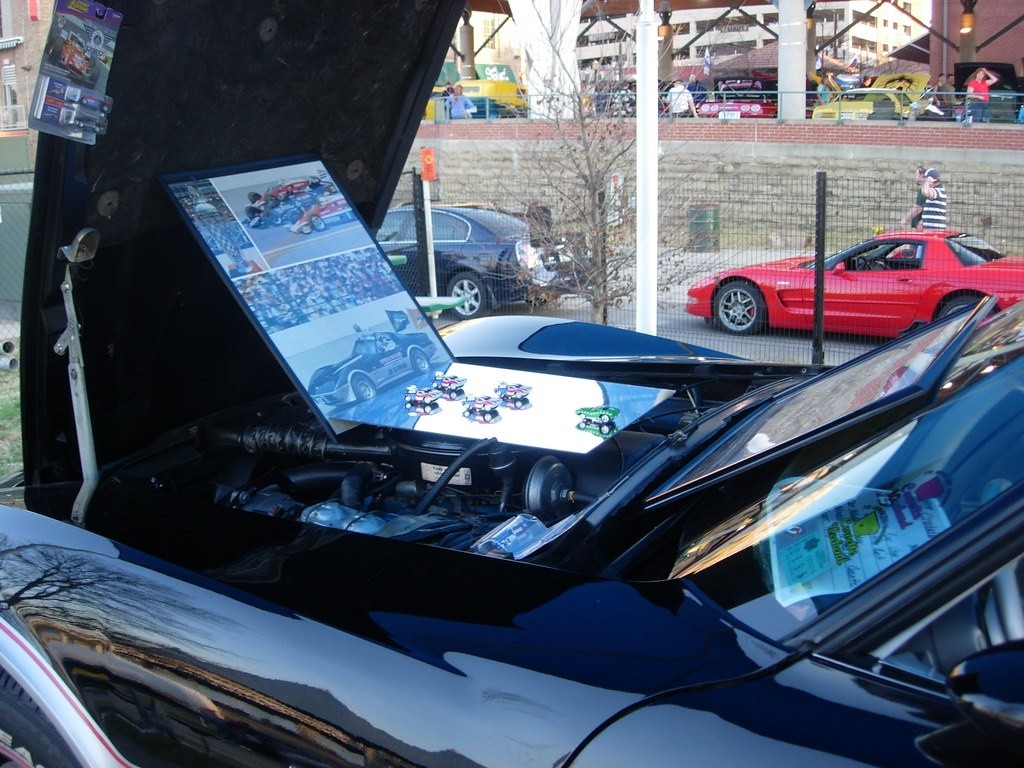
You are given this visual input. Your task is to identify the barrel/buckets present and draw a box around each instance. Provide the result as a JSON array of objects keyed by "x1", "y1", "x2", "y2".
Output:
[{"x1": 687, "y1": 204, "x2": 719, "y2": 252}]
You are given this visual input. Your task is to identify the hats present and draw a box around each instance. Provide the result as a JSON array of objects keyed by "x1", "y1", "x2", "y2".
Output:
[{"x1": 925, "y1": 168, "x2": 940, "y2": 182}]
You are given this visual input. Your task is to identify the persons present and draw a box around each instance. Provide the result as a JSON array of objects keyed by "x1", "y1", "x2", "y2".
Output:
[
  {"x1": 915, "y1": 168, "x2": 947, "y2": 258},
  {"x1": 966, "y1": 68, "x2": 998, "y2": 123},
  {"x1": 614, "y1": 82, "x2": 636, "y2": 118},
  {"x1": 933, "y1": 73, "x2": 962, "y2": 106},
  {"x1": 895, "y1": 167, "x2": 927, "y2": 257},
  {"x1": 855, "y1": 76, "x2": 871, "y2": 100},
  {"x1": 664, "y1": 81, "x2": 698, "y2": 118},
  {"x1": 817, "y1": 76, "x2": 830, "y2": 106},
  {"x1": 440, "y1": 82, "x2": 473, "y2": 119}
]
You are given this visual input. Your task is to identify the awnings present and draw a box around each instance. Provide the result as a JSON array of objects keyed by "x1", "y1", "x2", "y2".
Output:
[{"x1": 886, "y1": 32, "x2": 930, "y2": 64}]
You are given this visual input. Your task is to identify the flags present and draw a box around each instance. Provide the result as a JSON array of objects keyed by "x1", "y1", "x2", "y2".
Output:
[
  {"x1": 815, "y1": 45, "x2": 822, "y2": 70},
  {"x1": 849, "y1": 54, "x2": 860, "y2": 69},
  {"x1": 703, "y1": 46, "x2": 711, "y2": 76}
]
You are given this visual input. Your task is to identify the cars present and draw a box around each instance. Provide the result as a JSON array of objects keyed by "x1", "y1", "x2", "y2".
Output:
[
  {"x1": 686, "y1": 232, "x2": 1024, "y2": 345},
  {"x1": 695, "y1": 76, "x2": 816, "y2": 121},
  {"x1": 371, "y1": 198, "x2": 599, "y2": 321},
  {"x1": 464, "y1": 95, "x2": 518, "y2": 120},
  {"x1": 915, "y1": 61, "x2": 1024, "y2": 123},
  {"x1": 1, "y1": 0, "x2": 1024, "y2": 768},
  {"x1": 811, "y1": 72, "x2": 935, "y2": 123}
]
